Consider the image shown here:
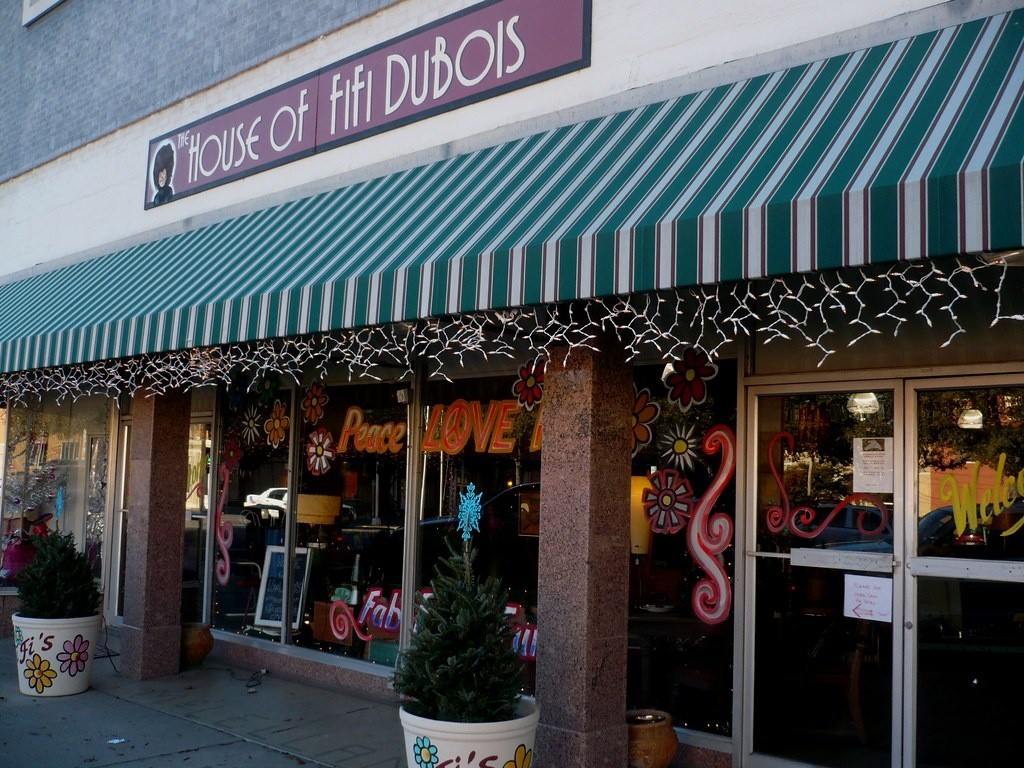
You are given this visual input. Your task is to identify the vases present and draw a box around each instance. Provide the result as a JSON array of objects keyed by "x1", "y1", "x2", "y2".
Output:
[
  {"x1": 625, "y1": 709, "x2": 681, "y2": 768},
  {"x1": 181, "y1": 622, "x2": 215, "y2": 666}
]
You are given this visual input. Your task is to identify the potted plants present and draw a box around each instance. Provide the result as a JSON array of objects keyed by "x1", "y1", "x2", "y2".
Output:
[
  {"x1": 11, "y1": 528, "x2": 104, "y2": 697},
  {"x1": 387, "y1": 535, "x2": 541, "y2": 768}
]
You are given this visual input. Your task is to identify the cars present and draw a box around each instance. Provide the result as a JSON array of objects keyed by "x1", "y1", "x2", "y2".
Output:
[
  {"x1": 242, "y1": 488, "x2": 291, "y2": 518},
  {"x1": 305, "y1": 481, "x2": 546, "y2": 631},
  {"x1": 631, "y1": 496, "x2": 1023, "y2": 660}
]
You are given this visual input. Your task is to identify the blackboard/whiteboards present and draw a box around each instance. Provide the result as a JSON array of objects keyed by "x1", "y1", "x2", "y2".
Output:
[{"x1": 253, "y1": 545, "x2": 313, "y2": 630}]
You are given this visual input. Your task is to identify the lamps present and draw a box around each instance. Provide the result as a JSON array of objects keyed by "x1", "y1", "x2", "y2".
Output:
[
  {"x1": 847, "y1": 392, "x2": 880, "y2": 422},
  {"x1": 957, "y1": 409, "x2": 983, "y2": 429}
]
